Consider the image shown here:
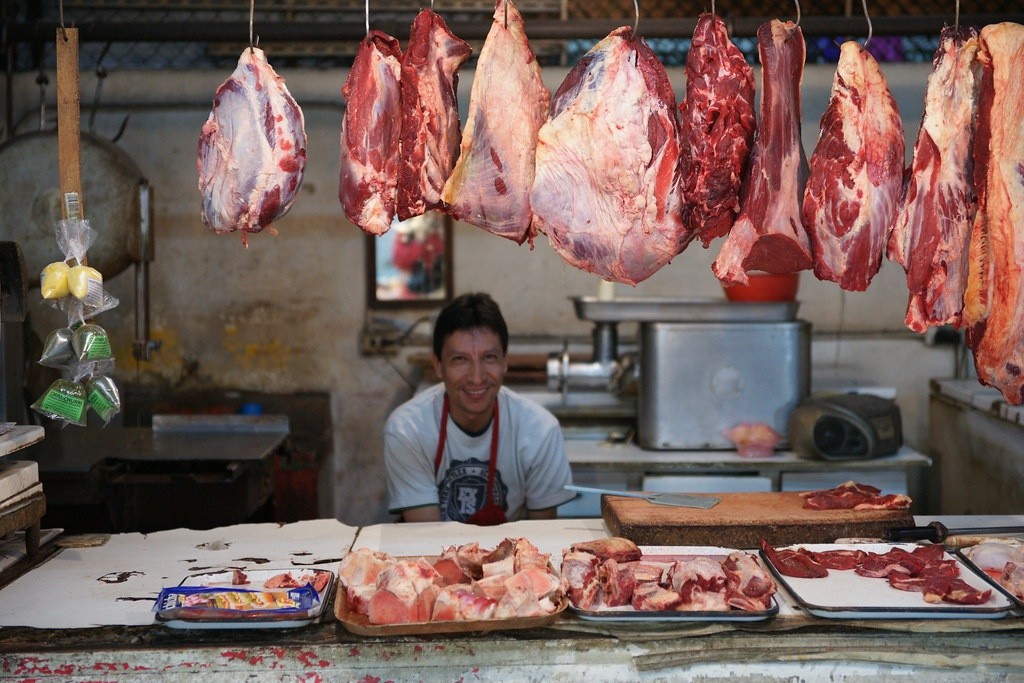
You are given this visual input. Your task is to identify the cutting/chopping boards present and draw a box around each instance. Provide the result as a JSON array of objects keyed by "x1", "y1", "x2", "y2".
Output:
[{"x1": 601, "y1": 491, "x2": 915, "y2": 548}]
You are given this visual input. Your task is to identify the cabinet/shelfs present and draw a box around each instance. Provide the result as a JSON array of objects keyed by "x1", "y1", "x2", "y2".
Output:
[{"x1": 927, "y1": 377, "x2": 1024, "y2": 517}]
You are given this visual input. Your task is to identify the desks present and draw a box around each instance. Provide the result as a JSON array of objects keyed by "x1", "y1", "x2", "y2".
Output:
[{"x1": 561, "y1": 443, "x2": 934, "y2": 493}]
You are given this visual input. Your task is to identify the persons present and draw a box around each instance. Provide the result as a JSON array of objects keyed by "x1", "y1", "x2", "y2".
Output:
[{"x1": 383, "y1": 292, "x2": 577, "y2": 523}]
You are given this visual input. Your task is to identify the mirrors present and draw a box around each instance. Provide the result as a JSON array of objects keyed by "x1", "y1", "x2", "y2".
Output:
[{"x1": 366, "y1": 209, "x2": 452, "y2": 305}]
